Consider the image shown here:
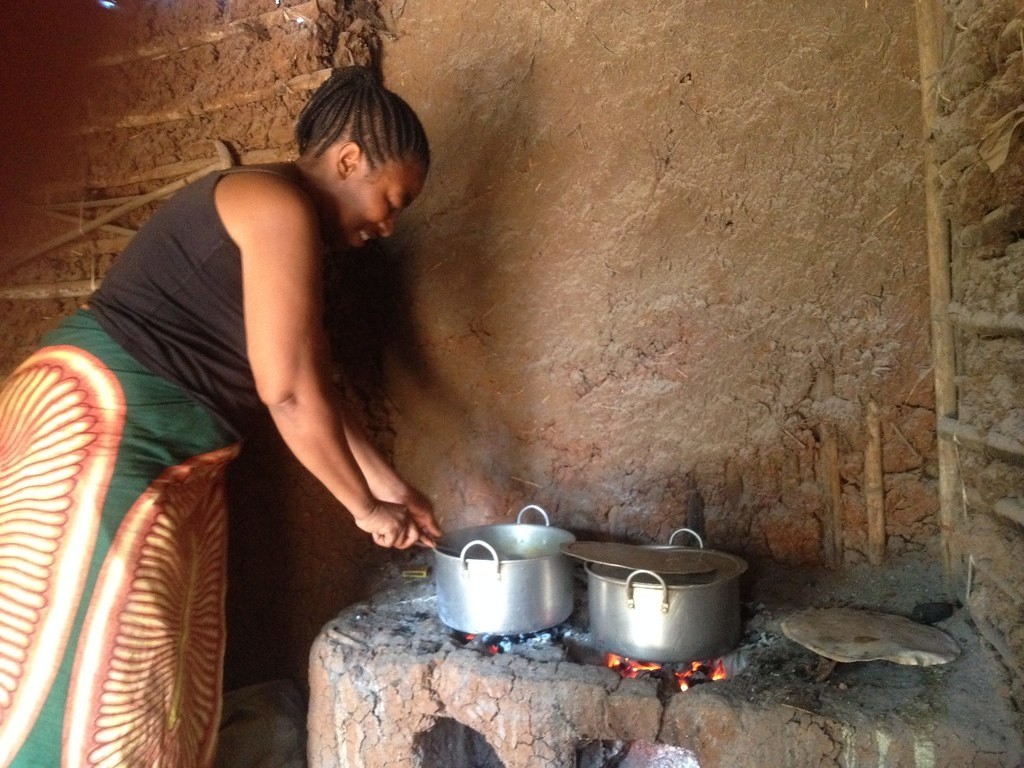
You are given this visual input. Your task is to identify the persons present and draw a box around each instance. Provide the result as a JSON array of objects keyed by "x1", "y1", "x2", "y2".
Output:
[{"x1": 0, "y1": 65, "x2": 442, "y2": 768}]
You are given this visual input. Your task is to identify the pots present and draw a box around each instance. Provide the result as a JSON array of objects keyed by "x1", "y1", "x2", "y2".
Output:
[
  {"x1": 431, "y1": 505, "x2": 577, "y2": 635},
  {"x1": 560, "y1": 529, "x2": 749, "y2": 662}
]
